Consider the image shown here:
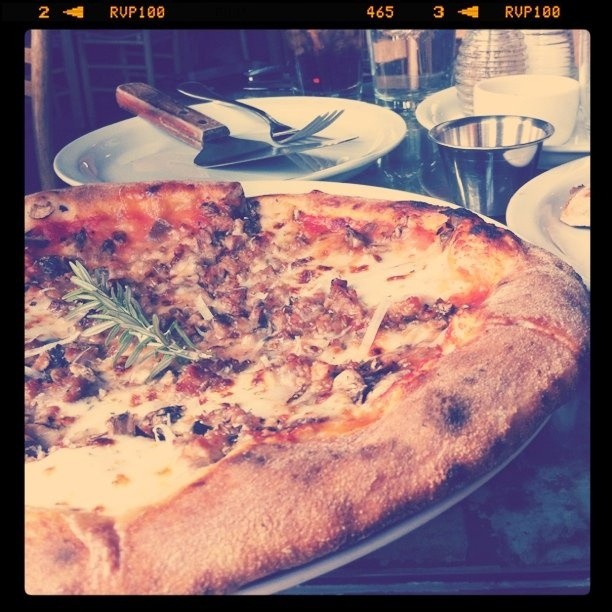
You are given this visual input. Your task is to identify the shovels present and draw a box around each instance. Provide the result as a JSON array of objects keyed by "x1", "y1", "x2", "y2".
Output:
[{"x1": 115, "y1": 80, "x2": 360, "y2": 167}]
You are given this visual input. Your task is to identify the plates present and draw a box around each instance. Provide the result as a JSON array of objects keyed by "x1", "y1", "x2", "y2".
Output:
[
  {"x1": 52, "y1": 98, "x2": 408, "y2": 192},
  {"x1": 414, "y1": 85, "x2": 589, "y2": 154},
  {"x1": 500, "y1": 157, "x2": 590, "y2": 299}
]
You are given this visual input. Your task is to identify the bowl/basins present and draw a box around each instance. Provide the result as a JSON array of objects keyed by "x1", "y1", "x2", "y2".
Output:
[
  {"x1": 455, "y1": 28, "x2": 514, "y2": 117},
  {"x1": 429, "y1": 115, "x2": 552, "y2": 214},
  {"x1": 477, "y1": 75, "x2": 578, "y2": 145},
  {"x1": 529, "y1": 31, "x2": 573, "y2": 80}
]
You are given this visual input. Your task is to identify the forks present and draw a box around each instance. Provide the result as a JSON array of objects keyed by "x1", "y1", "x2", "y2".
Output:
[{"x1": 177, "y1": 81, "x2": 347, "y2": 145}]
